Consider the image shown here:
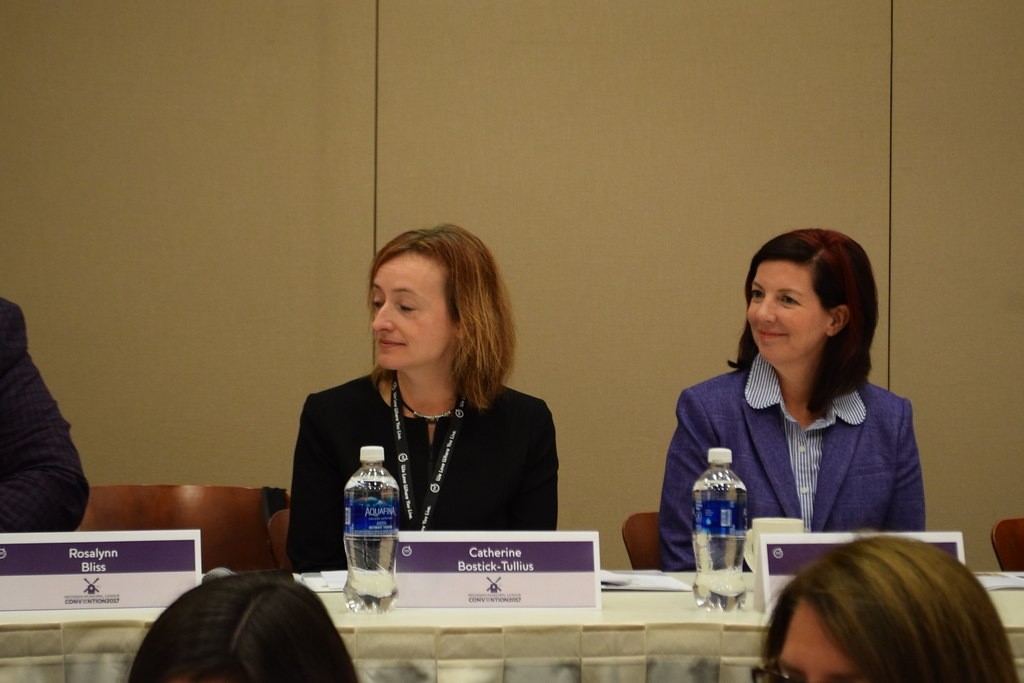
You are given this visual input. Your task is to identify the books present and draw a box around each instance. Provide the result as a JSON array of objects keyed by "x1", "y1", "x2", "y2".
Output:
[
  {"x1": 968, "y1": 571, "x2": 1023, "y2": 594},
  {"x1": 599, "y1": 567, "x2": 691, "y2": 591},
  {"x1": 291, "y1": 568, "x2": 349, "y2": 595}
]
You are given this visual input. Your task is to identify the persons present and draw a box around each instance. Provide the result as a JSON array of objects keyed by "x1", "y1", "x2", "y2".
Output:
[
  {"x1": 760, "y1": 533, "x2": 1018, "y2": 683},
  {"x1": 126, "y1": 567, "x2": 364, "y2": 683},
  {"x1": 285, "y1": 222, "x2": 560, "y2": 572},
  {"x1": 656, "y1": 228, "x2": 926, "y2": 576},
  {"x1": 0, "y1": 296, "x2": 93, "y2": 537}
]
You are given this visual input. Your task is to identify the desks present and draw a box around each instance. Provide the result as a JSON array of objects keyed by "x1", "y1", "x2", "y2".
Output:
[{"x1": 0, "y1": 572, "x2": 1024, "y2": 683}]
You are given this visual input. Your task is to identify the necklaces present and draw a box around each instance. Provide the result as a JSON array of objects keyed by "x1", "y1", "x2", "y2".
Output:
[{"x1": 396, "y1": 390, "x2": 457, "y2": 423}]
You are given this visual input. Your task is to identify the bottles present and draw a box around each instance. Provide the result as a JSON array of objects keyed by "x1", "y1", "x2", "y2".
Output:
[
  {"x1": 691, "y1": 448, "x2": 748, "y2": 610},
  {"x1": 343, "y1": 445, "x2": 398, "y2": 612}
]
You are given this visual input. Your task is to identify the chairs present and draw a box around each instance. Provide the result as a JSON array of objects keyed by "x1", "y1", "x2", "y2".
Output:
[{"x1": 80, "y1": 484, "x2": 290, "y2": 574}]
